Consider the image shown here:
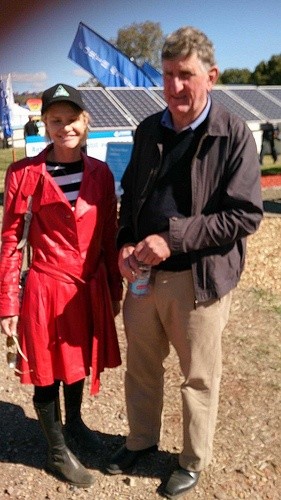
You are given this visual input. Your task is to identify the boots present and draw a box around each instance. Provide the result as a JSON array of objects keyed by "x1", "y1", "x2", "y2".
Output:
[
  {"x1": 62, "y1": 382, "x2": 109, "y2": 457},
  {"x1": 32, "y1": 396, "x2": 95, "y2": 487}
]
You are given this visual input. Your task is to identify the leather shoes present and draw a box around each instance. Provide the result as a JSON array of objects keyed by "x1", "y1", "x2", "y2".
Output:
[
  {"x1": 105, "y1": 442, "x2": 158, "y2": 474},
  {"x1": 162, "y1": 468, "x2": 194, "y2": 496}
]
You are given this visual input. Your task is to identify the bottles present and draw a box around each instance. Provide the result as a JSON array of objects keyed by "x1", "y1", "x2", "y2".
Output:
[{"x1": 129, "y1": 259, "x2": 151, "y2": 298}]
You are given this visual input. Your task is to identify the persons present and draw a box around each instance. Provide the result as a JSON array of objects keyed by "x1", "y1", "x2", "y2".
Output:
[
  {"x1": 105, "y1": 26, "x2": 264, "y2": 500},
  {"x1": 24, "y1": 115, "x2": 39, "y2": 139},
  {"x1": 0, "y1": 82, "x2": 123, "y2": 489}
]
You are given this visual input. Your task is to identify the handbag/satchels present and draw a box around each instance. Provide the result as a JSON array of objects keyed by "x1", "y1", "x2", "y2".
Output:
[{"x1": 19, "y1": 270, "x2": 27, "y2": 299}]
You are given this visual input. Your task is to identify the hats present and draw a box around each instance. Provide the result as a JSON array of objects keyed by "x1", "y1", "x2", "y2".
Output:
[{"x1": 41, "y1": 83, "x2": 85, "y2": 115}]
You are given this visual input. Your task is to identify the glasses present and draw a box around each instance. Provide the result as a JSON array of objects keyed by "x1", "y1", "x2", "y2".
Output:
[{"x1": 6, "y1": 336, "x2": 34, "y2": 374}]
